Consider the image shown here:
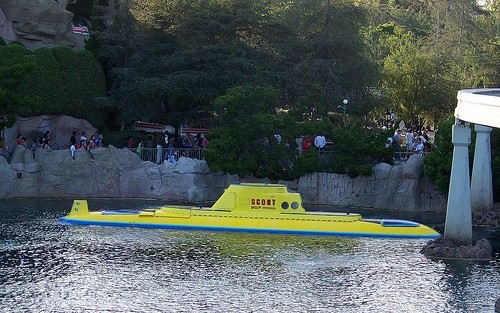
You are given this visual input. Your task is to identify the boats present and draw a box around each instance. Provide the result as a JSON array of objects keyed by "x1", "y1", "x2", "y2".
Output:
[{"x1": 57, "y1": 181, "x2": 443, "y2": 240}]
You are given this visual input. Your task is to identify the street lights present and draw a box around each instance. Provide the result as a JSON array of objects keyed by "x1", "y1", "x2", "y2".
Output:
[{"x1": 342, "y1": 99, "x2": 349, "y2": 124}]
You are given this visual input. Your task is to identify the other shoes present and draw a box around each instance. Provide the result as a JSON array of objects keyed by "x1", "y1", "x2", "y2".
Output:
[
  {"x1": 404, "y1": 157, "x2": 407, "y2": 160},
  {"x1": 392, "y1": 157, "x2": 396, "y2": 160},
  {"x1": 400, "y1": 157, "x2": 403, "y2": 160}
]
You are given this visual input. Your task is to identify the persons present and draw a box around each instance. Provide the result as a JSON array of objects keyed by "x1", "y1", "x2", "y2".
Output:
[
  {"x1": 393, "y1": 124, "x2": 430, "y2": 158},
  {"x1": 0, "y1": 130, "x2": 53, "y2": 158},
  {"x1": 127, "y1": 131, "x2": 208, "y2": 163},
  {"x1": 69, "y1": 131, "x2": 104, "y2": 159},
  {"x1": 302, "y1": 133, "x2": 326, "y2": 154}
]
